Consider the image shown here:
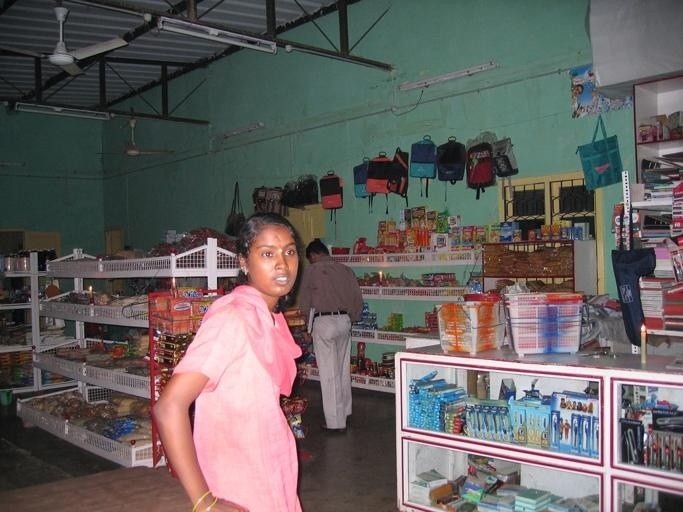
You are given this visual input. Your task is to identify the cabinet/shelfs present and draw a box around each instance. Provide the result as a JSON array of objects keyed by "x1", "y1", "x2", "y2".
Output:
[
  {"x1": 584, "y1": 0, "x2": 683, "y2": 278},
  {"x1": 0, "y1": 237, "x2": 576, "y2": 467},
  {"x1": 394, "y1": 340, "x2": 681, "y2": 509}
]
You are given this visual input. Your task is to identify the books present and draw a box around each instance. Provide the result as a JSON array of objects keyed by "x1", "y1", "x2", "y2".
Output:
[{"x1": 636, "y1": 226, "x2": 683, "y2": 334}]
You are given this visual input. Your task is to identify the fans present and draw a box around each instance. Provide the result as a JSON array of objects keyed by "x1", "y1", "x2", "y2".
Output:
[{"x1": 0, "y1": 7, "x2": 128, "y2": 76}]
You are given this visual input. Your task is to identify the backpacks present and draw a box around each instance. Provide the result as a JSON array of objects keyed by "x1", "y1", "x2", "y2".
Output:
[
  {"x1": 252, "y1": 178, "x2": 318, "y2": 217},
  {"x1": 409, "y1": 140, "x2": 437, "y2": 179},
  {"x1": 320, "y1": 174, "x2": 343, "y2": 209},
  {"x1": 353, "y1": 161, "x2": 375, "y2": 198},
  {"x1": 465, "y1": 141, "x2": 496, "y2": 188},
  {"x1": 363, "y1": 156, "x2": 390, "y2": 194},
  {"x1": 491, "y1": 138, "x2": 519, "y2": 177},
  {"x1": 385, "y1": 149, "x2": 410, "y2": 196},
  {"x1": 435, "y1": 141, "x2": 466, "y2": 181}
]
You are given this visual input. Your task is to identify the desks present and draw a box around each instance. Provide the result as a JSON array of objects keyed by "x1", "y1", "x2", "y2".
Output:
[{"x1": 0, "y1": 467, "x2": 195, "y2": 512}]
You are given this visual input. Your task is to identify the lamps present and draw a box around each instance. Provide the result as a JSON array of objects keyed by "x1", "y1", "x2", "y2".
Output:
[
  {"x1": 157, "y1": 14, "x2": 277, "y2": 55},
  {"x1": 14, "y1": 101, "x2": 110, "y2": 121},
  {"x1": 97, "y1": 118, "x2": 173, "y2": 157}
]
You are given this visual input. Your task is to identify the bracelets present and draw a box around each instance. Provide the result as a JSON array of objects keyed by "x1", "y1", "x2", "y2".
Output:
[
  {"x1": 204, "y1": 496, "x2": 218, "y2": 512},
  {"x1": 191, "y1": 489, "x2": 212, "y2": 512}
]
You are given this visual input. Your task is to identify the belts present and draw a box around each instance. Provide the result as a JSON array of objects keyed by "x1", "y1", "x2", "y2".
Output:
[{"x1": 314, "y1": 310, "x2": 347, "y2": 318}]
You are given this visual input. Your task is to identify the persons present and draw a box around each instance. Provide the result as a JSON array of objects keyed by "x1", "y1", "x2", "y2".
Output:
[
  {"x1": 154, "y1": 211, "x2": 306, "y2": 511},
  {"x1": 570, "y1": 84, "x2": 583, "y2": 116},
  {"x1": 297, "y1": 238, "x2": 364, "y2": 434}
]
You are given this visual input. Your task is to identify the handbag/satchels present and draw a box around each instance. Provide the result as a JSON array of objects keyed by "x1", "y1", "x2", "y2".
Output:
[
  {"x1": 611, "y1": 204, "x2": 656, "y2": 344},
  {"x1": 576, "y1": 112, "x2": 625, "y2": 191},
  {"x1": 225, "y1": 212, "x2": 246, "y2": 237}
]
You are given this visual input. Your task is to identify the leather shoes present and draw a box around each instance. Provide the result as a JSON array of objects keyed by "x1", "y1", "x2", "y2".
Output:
[{"x1": 321, "y1": 423, "x2": 347, "y2": 434}]
